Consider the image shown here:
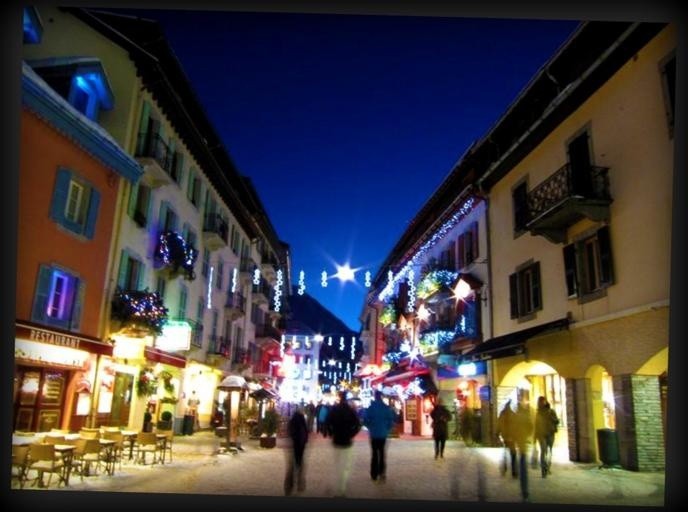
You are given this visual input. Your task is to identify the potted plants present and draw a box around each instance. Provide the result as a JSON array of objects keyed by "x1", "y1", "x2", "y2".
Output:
[
  {"x1": 260, "y1": 407, "x2": 278, "y2": 448},
  {"x1": 143, "y1": 412, "x2": 153, "y2": 432},
  {"x1": 158, "y1": 411, "x2": 172, "y2": 430}
]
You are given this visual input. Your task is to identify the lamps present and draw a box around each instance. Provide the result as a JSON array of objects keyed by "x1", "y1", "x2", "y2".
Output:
[{"x1": 454, "y1": 273, "x2": 487, "y2": 306}]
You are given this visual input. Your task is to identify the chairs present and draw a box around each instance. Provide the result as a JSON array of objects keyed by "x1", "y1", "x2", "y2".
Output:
[{"x1": 12, "y1": 425, "x2": 173, "y2": 489}]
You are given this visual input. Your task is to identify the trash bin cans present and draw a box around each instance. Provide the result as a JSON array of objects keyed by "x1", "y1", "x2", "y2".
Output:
[
  {"x1": 597, "y1": 429, "x2": 621, "y2": 468},
  {"x1": 472, "y1": 414, "x2": 482, "y2": 442}
]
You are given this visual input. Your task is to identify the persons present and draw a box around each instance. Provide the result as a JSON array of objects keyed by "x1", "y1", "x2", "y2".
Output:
[{"x1": 282, "y1": 387, "x2": 559, "y2": 497}]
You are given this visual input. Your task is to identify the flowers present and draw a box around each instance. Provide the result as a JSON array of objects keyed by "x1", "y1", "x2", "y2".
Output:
[{"x1": 137, "y1": 367, "x2": 157, "y2": 395}]
